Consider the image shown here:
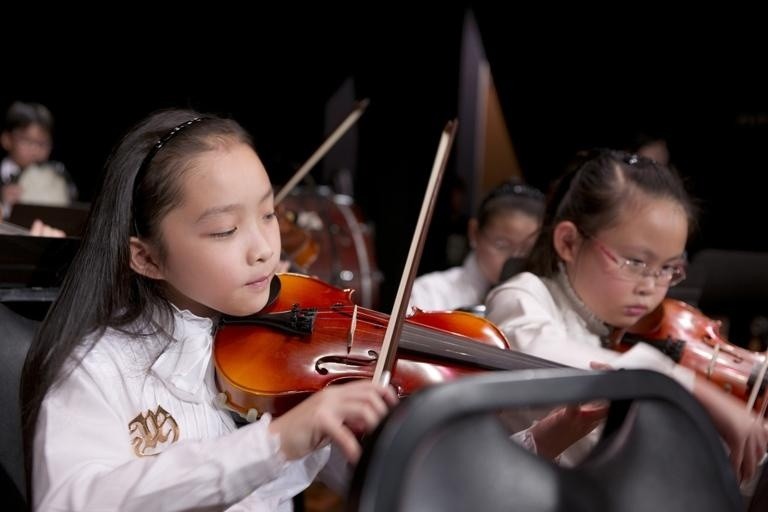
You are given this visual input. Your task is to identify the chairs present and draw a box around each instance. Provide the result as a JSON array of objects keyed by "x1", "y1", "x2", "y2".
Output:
[
  {"x1": 0, "y1": 286, "x2": 64, "y2": 511},
  {"x1": 346, "y1": 369, "x2": 746, "y2": 512}
]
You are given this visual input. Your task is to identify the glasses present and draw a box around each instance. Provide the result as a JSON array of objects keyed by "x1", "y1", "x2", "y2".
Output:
[
  {"x1": 577, "y1": 226, "x2": 688, "y2": 288},
  {"x1": 479, "y1": 225, "x2": 532, "y2": 258}
]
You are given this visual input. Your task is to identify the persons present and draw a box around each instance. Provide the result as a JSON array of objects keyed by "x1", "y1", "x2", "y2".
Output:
[
  {"x1": 24, "y1": 108, "x2": 630, "y2": 510},
  {"x1": 1, "y1": 99, "x2": 81, "y2": 237},
  {"x1": 468, "y1": 144, "x2": 768, "y2": 491},
  {"x1": 404, "y1": 177, "x2": 549, "y2": 314}
]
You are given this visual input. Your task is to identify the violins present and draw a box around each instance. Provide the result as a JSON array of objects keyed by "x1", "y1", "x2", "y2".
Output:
[
  {"x1": 611, "y1": 297, "x2": 764, "y2": 412},
  {"x1": 211, "y1": 273, "x2": 604, "y2": 418}
]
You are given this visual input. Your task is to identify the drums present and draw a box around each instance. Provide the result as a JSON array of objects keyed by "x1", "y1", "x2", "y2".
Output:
[{"x1": 272, "y1": 187, "x2": 383, "y2": 312}]
